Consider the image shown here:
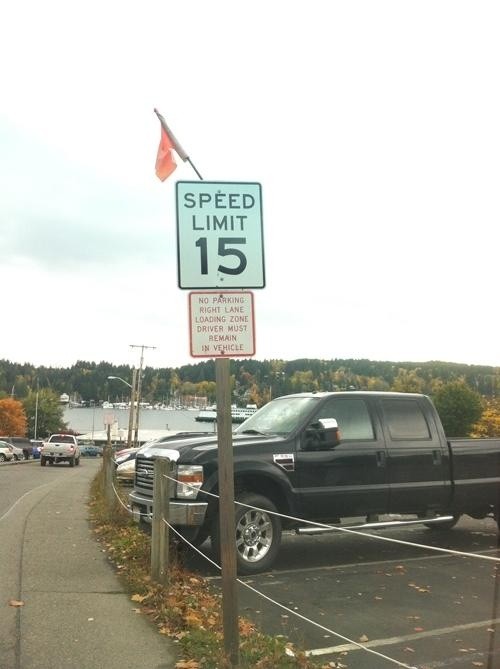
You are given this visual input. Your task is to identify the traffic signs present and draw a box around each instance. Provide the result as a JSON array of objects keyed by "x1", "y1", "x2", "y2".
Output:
[{"x1": 176, "y1": 180, "x2": 264, "y2": 289}]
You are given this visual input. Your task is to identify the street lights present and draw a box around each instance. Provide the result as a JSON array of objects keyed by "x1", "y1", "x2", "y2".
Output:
[
  {"x1": 106, "y1": 375, "x2": 138, "y2": 446},
  {"x1": 90, "y1": 399, "x2": 95, "y2": 442},
  {"x1": 264, "y1": 375, "x2": 272, "y2": 401},
  {"x1": 34, "y1": 377, "x2": 39, "y2": 439}
]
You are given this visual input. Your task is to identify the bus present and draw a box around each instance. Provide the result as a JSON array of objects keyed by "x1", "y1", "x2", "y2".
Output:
[{"x1": 30, "y1": 439, "x2": 45, "y2": 459}]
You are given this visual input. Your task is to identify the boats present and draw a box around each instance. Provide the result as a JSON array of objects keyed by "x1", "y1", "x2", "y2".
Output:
[
  {"x1": 195, "y1": 401, "x2": 258, "y2": 423},
  {"x1": 60, "y1": 393, "x2": 69, "y2": 403}
]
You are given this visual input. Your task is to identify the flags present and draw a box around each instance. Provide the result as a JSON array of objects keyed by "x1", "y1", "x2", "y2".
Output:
[{"x1": 154, "y1": 126, "x2": 176, "y2": 182}]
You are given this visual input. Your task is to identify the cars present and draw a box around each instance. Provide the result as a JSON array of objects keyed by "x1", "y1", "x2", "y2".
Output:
[
  {"x1": 80, "y1": 446, "x2": 103, "y2": 458},
  {"x1": 0, "y1": 440, "x2": 14, "y2": 462},
  {"x1": 3, "y1": 441, "x2": 24, "y2": 461},
  {"x1": 114, "y1": 430, "x2": 217, "y2": 481}
]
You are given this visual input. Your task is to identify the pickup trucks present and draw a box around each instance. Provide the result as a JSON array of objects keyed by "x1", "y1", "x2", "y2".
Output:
[
  {"x1": 126, "y1": 391, "x2": 500, "y2": 576},
  {"x1": 40, "y1": 433, "x2": 81, "y2": 467}
]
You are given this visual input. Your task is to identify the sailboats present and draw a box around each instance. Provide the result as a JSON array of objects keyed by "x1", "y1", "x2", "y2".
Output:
[
  {"x1": 101, "y1": 392, "x2": 200, "y2": 411},
  {"x1": 71, "y1": 391, "x2": 86, "y2": 408}
]
